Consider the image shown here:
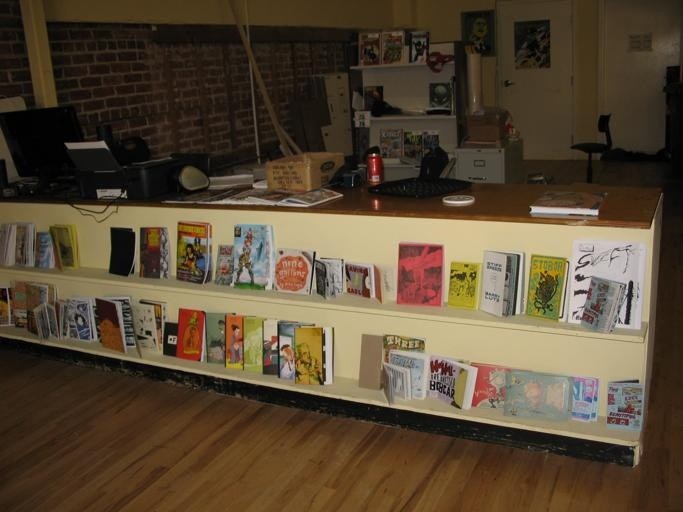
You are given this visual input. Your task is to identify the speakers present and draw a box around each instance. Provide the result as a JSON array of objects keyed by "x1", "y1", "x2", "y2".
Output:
[{"x1": 0, "y1": 159, "x2": 9, "y2": 188}]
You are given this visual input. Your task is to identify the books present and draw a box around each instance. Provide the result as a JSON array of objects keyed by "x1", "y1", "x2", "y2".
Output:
[
  {"x1": 161, "y1": 189, "x2": 343, "y2": 208},
  {"x1": 529, "y1": 190, "x2": 608, "y2": 216},
  {"x1": 358, "y1": 29, "x2": 429, "y2": 65},
  {"x1": 380, "y1": 128, "x2": 439, "y2": 161}
]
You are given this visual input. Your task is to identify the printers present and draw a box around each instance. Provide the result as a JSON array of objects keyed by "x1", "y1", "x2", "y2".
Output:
[{"x1": 69, "y1": 148, "x2": 210, "y2": 199}]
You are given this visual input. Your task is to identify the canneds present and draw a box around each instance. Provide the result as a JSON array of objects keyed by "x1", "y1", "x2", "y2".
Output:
[{"x1": 367, "y1": 153, "x2": 384, "y2": 185}]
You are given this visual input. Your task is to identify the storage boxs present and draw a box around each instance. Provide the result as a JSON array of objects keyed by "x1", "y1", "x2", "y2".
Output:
[{"x1": 265, "y1": 151, "x2": 345, "y2": 196}]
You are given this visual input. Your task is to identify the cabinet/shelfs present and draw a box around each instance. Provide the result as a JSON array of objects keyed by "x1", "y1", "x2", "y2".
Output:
[
  {"x1": 0, "y1": 265, "x2": 648, "y2": 447},
  {"x1": 455, "y1": 140, "x2": 523, "y2": 184},
  {"x1": 350, "y1": 41, "x2": 466, "y2": 182}
]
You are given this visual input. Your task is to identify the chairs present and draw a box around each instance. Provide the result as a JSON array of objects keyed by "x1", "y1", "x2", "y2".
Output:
[{"x1": 570, "y1": 114, "x2": 612, "y2": 185}]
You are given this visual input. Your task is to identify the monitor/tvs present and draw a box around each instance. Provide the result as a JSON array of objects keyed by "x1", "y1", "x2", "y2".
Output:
[{"x1": 0, "y1": 105, "x2": 85, "y2": 199}]
